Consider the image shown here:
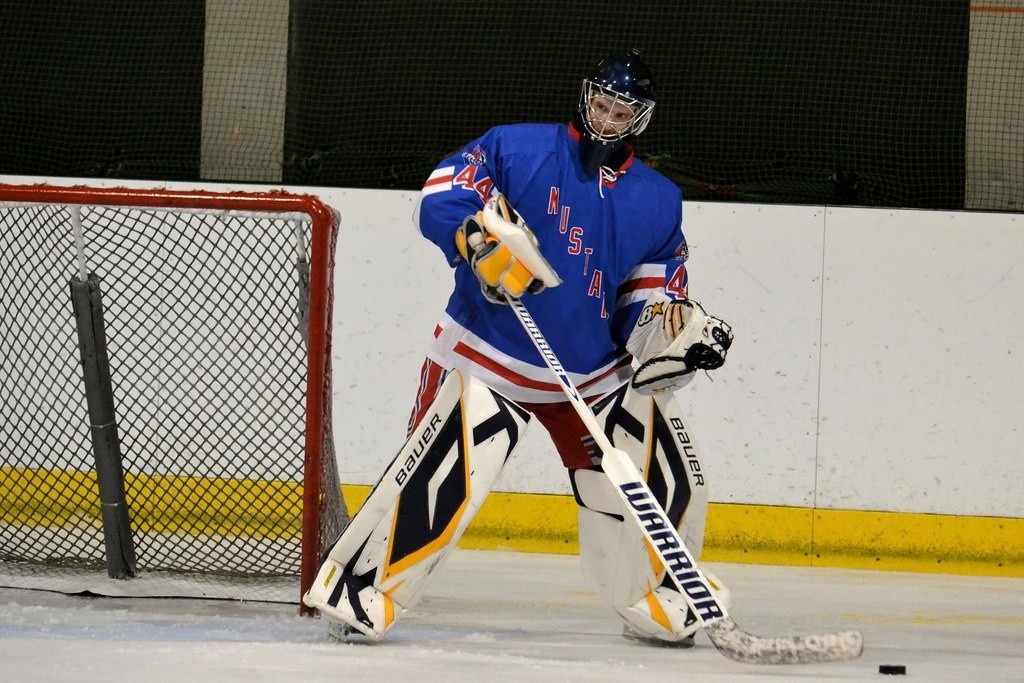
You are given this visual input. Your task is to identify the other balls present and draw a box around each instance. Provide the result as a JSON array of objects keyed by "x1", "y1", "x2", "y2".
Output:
[{"x1": 880, "y1": 664, "x2": 906, "y2": 675}]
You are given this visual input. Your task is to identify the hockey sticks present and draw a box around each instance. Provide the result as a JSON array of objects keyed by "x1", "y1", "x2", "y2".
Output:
[{"x1": 469, "y1": 230, "x2": 865, "y2": 665}]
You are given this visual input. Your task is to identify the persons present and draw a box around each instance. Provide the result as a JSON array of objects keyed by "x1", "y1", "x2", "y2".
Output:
[{"x1": 303, "y1": 45, "x2": 732, "y2": 641}]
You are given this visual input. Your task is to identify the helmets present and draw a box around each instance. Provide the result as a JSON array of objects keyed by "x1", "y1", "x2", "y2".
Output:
[{"x1": 575, "y1": 47, "x2": 657, "y2": 171}]
